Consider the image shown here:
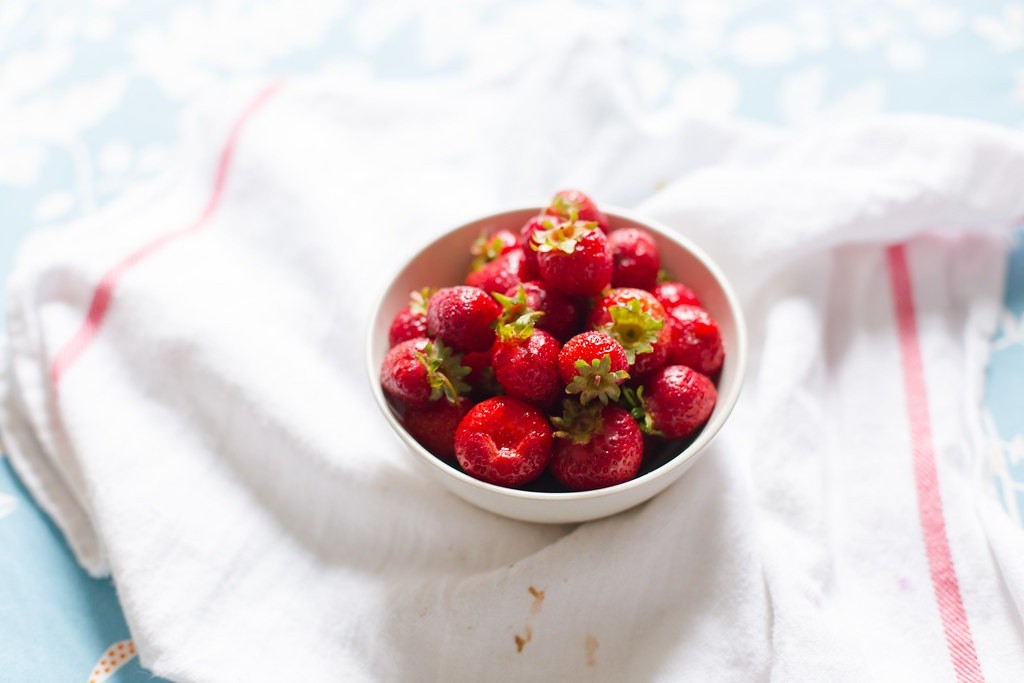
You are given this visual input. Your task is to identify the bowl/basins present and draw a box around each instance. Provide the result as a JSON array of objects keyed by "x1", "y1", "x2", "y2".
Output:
[{"x1": 363, "y1": 208, "x2": 750, "y2": 526}]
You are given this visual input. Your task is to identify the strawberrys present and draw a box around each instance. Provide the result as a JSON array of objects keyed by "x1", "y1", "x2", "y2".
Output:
[{"x1": 378, "y1": 188, "x2": 726, "y2": 490}]
